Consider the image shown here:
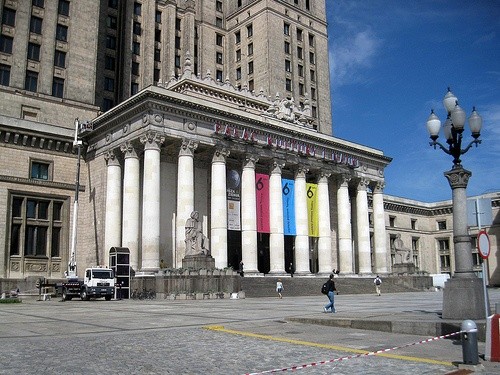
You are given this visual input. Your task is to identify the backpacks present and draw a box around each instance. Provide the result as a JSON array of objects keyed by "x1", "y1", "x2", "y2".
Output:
[
  {"x1": 321, "y1": 282, "x2": 329, "y2": 294},
  {"x1": 376, "y1": 278, "x2": 380, "y2": 285}
]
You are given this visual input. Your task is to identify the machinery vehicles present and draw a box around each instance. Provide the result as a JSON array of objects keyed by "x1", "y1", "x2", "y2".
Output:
[{"x1": 36, "y1": 117, "x2": 116, "y2": 302}]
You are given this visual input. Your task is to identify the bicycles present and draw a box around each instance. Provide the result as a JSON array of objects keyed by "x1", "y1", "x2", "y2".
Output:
[{"x1": 131, "y1": 288, "x2": 156, "y2": 300}]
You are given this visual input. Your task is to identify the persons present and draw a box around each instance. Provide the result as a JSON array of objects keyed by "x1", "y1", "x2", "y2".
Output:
[
  {"x1": 373, "y1": 275, "x2": 382, "y2": 296},
  {"x1": 321, "y1": 274, "x2": 338, "y2": 313},
  {"x1": 238, "y1": 260, "x2": 245, "y2": 278},
  {"x1": 289, "y1": 263, "x2": 293, "y2": 278},
  {"x1": 184, "y1": 211, "x2": 209, "y2": 256},
  {"x1": 276, "y1": 278, "x2": 284, "y2": 299},
  {"x1": 392, "y1": 233, "x2": 413, "y2": 263}
]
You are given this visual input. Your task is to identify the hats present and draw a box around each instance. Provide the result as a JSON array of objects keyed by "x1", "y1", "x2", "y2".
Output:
[{"x1": 277, "y1": 278, "x2": 281, "y2": 281}]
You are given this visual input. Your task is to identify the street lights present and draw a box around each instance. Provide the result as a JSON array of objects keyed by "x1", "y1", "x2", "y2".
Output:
[{"x1": 425, "y1": 87, "x2": 492, "y2": 319}]
